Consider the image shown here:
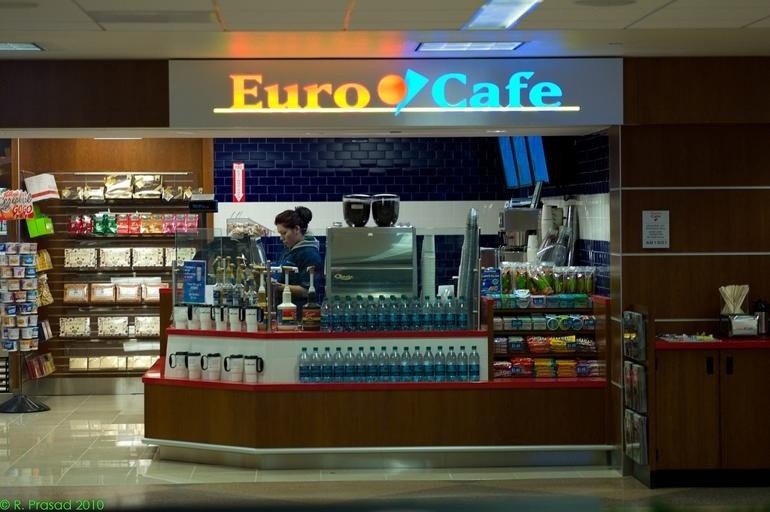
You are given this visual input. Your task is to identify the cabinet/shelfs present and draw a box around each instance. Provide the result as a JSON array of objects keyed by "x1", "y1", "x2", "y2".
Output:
[
  {"x1": 142, "y1": 324, "x2": 611, "y2": 396},
  {"x1": 35, "y1": 198, "x2": 221, "y2": 396},
  {"x1": 623, "y1": 325, "x2": 768, "y2": 494}
]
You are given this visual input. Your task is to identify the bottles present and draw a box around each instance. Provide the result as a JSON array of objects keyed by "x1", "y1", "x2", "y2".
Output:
[
  {"x1": 754, "y1": 297, "x2": 767, "y2": 335},
  {"x1": 342, "y1": 193, "x2": 401, "y2": 228},
  {"x1": 212, "y1": 255, "x2": 271, "y2": 331},
  {"x1": 275, "y1": 266, "x2": 480, "y2": 382}
]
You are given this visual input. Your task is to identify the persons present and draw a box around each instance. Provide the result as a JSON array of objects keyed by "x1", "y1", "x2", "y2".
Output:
[{"x1": 267, "y1": 205, "x2": 325, "y2": 309}]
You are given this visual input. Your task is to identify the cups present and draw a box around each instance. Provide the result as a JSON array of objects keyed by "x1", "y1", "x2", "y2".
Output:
[
  {"x1": 173, "y1": 303, "x2": 265, "y2": 333},
  {"x1": 167, "y1": 350, "x2": 264, "y2": 382}
]
